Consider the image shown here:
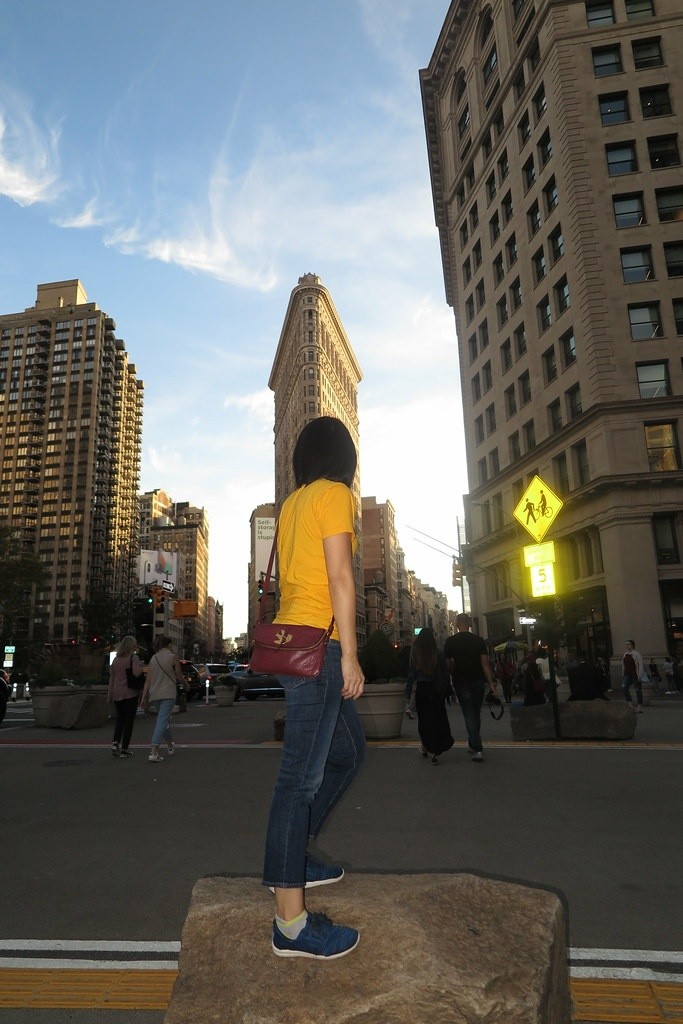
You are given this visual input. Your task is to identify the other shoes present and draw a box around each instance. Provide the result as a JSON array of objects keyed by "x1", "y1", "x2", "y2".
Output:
[
  {"x1": 472, "y1": 752, "x2": 483, "y2": 761},
  {"x1": 422, "y1": 750, "x2": 428, "y2": 759},
  {"x1": 432, "y1": 755, "x2": 440, "y2": 764},
  {"x1": 468, "y1": 747, "x2": 473, "y2": 753}
]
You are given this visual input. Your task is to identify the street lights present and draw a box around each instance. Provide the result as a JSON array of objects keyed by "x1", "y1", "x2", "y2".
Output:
[{"x1": 472, "y1": 500, "x2": 533, "y2": 652}]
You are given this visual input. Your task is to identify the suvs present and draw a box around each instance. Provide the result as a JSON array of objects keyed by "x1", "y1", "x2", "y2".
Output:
[{"x1": 179, "y1": 659, "x2": 204, "y2": 701}]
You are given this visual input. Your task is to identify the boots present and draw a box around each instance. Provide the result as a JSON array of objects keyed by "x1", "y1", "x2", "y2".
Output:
[
  {"x1": 636, "y1": 704, "x2": 642, "y2": 711},
  {"x1": 626, "y1": 701, "x2": 634, "y2": 710}
]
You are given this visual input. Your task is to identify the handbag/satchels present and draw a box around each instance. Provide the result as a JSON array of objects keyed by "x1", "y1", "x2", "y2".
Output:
[
  {"x1": 125, "y1": 655, "x2": 146, "y2": 690},
  {"x1": 175, "y1": 694, "x2": 184, "y2": 705},
  {"x1": 641, "y1": 671, "x2": 650, "y2": 683},
  {"x1": 533, "y1": 679, "x2": 545, "y2": 693},
  {"x1": 249, "y1": 624, "x2": 327, "y2": 676}
]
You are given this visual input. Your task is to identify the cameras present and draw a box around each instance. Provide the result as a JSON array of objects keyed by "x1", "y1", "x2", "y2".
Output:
[{"x1": 485, "y1": 690, "x2": 501, "y2": 705}]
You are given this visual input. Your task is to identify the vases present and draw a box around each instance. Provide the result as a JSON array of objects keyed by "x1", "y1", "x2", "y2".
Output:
[{"x1": 629, "y1": 680, "x2": 656, "y2": 705}]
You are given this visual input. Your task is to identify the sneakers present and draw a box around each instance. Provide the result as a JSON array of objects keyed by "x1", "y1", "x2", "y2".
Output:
[
  {"x1": 269, "y1": 857, "x2": 345, "y2": 894},
  {"x1": 149, "y1": 753, "x2": 164, "y2": 761},
  {"x1": 271, "y1": 910, "x2": 360, "y2": 960},
  {"x1": 120, "y1": 751, "x2": 133, "y2": 758},
  {"x1": 168, "y1": 742, "x2": 175, "y2": 755},
  {"x1": 112, "y1": 743, "x2": 119, "y2": 756}
]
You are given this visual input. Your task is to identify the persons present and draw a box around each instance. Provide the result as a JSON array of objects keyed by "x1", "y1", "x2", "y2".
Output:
[
  {"x1": 249, "y1": 416, "x2": 368, "y2": 962},
  {"x1": 403, "y1": 628, "x2": 453, "y2": 766},
  {"x1": 1, "y1": 670, "x2": 9, "y2": 725},
  {"x1": 444, "y1": 613, "x2": 500, "y2": 759},
  {"x1": 106, "y1": 635, "x2": 150, "y2": 759},
  {"x1": 139, "y1": 636, "x2": 191, "y2": 761},
  {"x1": 495, "y1": 640, "x2": 683, "y2": 714}
]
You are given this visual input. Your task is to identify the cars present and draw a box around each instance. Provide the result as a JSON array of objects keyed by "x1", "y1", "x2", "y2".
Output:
[
  {"x1": 218, "y1": 671, "x2": 284, "y2": 700},
  {"x1": 194, "y1": 661, "x2": 250, "y2": 696}
]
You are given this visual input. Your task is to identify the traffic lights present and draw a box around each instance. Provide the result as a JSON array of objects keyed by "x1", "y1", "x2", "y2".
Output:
[
  {"x1": 145, "y1": 586, "x2": 153, "y2": 607},
  {"x1": 156, "y1": 590, "x2": 165, "y2": 610},
  {"x1": 258, "y1": 580, "x2": 264, "y2": 595}
]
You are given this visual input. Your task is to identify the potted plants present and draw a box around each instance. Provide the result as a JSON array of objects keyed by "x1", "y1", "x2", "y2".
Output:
[
  {"x1": 29, "y1": 667, "x2": 114, "y2": 727},
  {"x1": 214, "y1": 675, "x2": 238, "y2": 707},
  {"x1": 550, "y1": 671, "x2": 571, "y2": 702},
  {"x1": 354, "y1": 631, "x2": 409, "y2": 739}
]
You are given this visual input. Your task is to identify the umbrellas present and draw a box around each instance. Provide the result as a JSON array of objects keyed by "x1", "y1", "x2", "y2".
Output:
[{"x1": 493, "y1": 640, "x2": 528, "y2": 652}]
